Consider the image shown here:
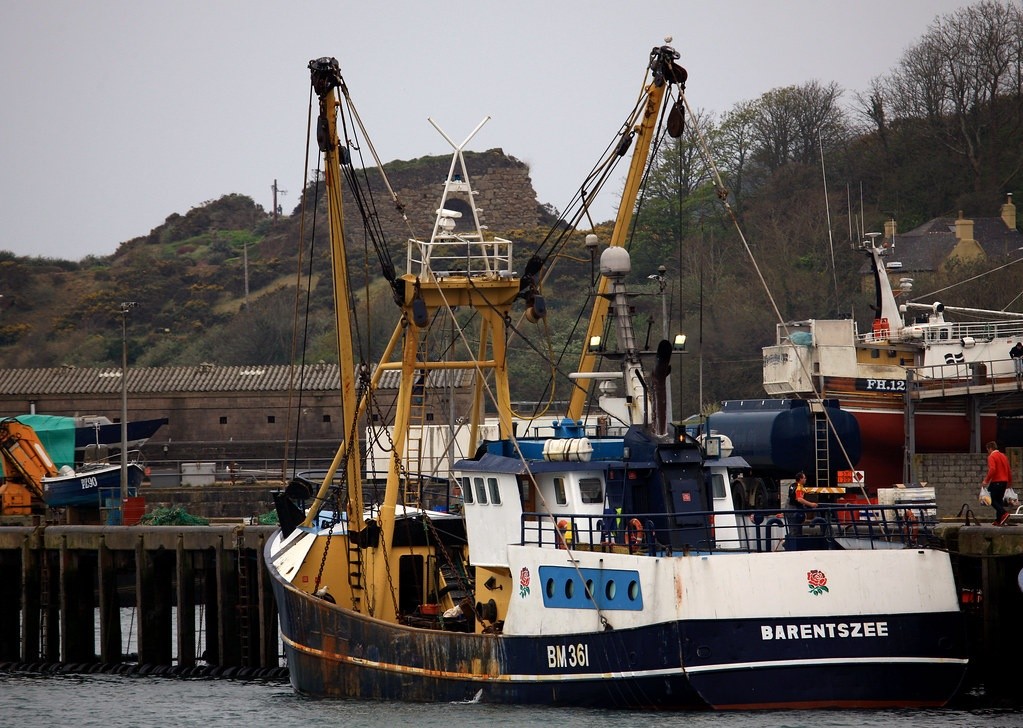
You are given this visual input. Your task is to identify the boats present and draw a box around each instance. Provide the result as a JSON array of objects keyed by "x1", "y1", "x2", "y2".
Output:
[
  {"x1": 264, "y1": 36, "x2": 969, "y2": 712},
  {"x1": 762, "y1": 180, "x2": 1023, "y2": 414},
  {"x1": 0, "y1": 413, "x2": 169, "y2": 507}
]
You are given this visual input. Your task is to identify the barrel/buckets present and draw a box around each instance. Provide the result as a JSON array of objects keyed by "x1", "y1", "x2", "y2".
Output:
[
  {"x1": 973, "y1": 364, "x2": 986, "y2": 385},
  {"x1": 106, "y1": 499, "x2": 121, "y2": 525},
  {"x1": 697, "y1": 432, "x2": 735, "y2": 459},
  {"x1": 122, "y1": 497, "x2": 145, "y2": 526},
  {"x1": 542, "y1": 437, "x2": 593, "y2": 461}
]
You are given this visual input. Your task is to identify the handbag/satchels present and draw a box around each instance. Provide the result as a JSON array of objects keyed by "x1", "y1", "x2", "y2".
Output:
[
  {"x1": 979, "y1": 487, "x2": 991, "y2": 507},
  {"x1": 1002, "y1": 488, "x2": 1019, "y2": 506}
]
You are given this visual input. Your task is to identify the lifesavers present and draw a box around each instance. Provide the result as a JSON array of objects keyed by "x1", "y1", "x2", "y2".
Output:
[
  {"x1": 558, "y1": 520, "x2": 572, "y2": 549},
  {"x1": 625, "y1": 518, "x2": 643, "y2": 553},
  {"x1": 903, "y1": 511, "x2": 918, "y2": 541}
]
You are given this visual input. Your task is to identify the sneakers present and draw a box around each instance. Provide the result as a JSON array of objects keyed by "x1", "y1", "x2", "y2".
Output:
[
  {"x1": 1000, "y1": 513, "x2": 1010, "y2": 524},
  {"x1": 991, "y1": 522, "x2": 1002, "y2": 527}
]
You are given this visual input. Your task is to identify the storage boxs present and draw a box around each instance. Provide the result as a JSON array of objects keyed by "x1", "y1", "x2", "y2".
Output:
[{"x1": 835, "y1": 497, "x2": 878, "y2": 521}]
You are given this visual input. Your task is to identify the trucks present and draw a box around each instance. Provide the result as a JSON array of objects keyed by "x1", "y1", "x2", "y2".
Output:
[{"x1": 686, "y1": 398, "x2": 862, "y2": 517}]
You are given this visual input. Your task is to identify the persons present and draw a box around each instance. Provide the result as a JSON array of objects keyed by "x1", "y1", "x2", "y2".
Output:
[
  {"x1": 782, "y1": 473, "x2": 818, "y2": 534},
  {"x1": 1009, "y1": 342, "x2": 1023, "y2": 375},
  {"x1": 981, "y1": 441, "x2": 1014, "y2": 526}
]
